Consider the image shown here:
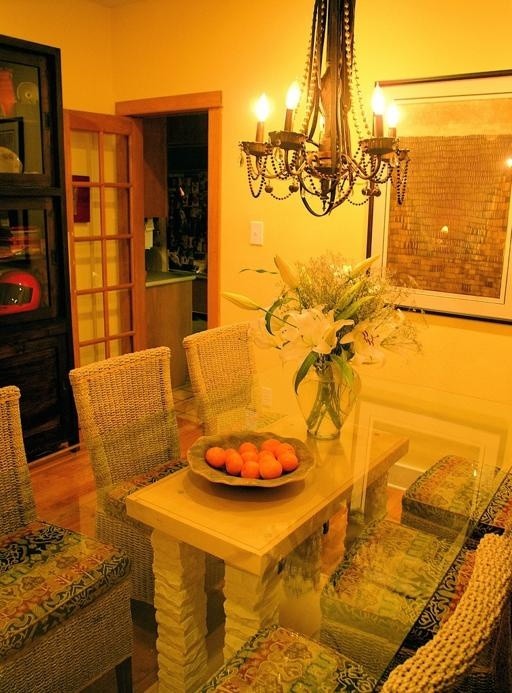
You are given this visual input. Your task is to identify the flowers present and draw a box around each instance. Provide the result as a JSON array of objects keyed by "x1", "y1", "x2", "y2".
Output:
[{"x1": 219, "y1": 247, "x2": 430, "y2": 434}]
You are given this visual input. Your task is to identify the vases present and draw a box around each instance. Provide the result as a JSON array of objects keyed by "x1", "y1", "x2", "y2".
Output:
[{"x1": 290, "y1": 353, "x2": 359, "y2": 440}]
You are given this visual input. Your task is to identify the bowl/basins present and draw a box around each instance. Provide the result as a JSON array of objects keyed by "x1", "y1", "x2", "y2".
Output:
[
  {"x1": 183, "y1": 469, "x2": 306, "y2": 511},
  {"x1": 186, "y1": 429, "x2": 316, "y2": 489}
]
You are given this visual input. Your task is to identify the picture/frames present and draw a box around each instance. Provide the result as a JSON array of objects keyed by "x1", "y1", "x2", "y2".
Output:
[
  {"x1": 343, "y1": 391, "x2": 508, "y2": 554},
  {"x1": 365, "y1": 69, "x2": 512, "y2": 325}
]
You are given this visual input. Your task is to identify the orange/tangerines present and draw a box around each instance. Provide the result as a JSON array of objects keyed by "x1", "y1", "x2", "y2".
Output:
[{"x1": 206, "y1": 439, "x2": 298, "y2": 480}]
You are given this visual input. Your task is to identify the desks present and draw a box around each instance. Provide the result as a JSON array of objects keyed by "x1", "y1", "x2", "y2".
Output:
[{"x1": 145, "y1": 267, "x2": 199, "y2": 390}]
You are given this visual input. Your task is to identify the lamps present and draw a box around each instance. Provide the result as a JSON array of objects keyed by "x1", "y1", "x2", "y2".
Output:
[{"x1": 237, "y1": 0, "x2": 412, "y2": 217}]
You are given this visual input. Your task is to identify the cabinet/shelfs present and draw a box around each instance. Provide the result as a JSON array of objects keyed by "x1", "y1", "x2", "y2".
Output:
[
  {"x1": 1, "y1": 338, "x2": 85, "y2": 458},
  {"x1": 0, "y1": 31, "x2": 72, "y2": 337},
  {"x1": 140, "y1": 116, "x2": 170, "y2": 218}
]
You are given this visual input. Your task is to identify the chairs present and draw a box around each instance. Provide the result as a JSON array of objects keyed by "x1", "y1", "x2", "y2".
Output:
[
  {"x1": 191, "y1": 532, "x2": 512, "y2": 692},
  {"x1": 68, "y1": 346, "x2": 226, "y2": 638},
  {"x1": 318, "y1": 510, "x2": 512, "y2": 692},
  {"x1": 399, "y1": 452, "x2": 512, "y2": 549},
  {"x1": 0, "y1": 384, "x2": 140, "y2": 693},
  {"x1": 181, "y1": 319, "x2": 285, "y2": 446}
]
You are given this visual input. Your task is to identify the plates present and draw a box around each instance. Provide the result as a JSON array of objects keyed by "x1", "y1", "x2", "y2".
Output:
[{"x1": 1, "y1": 146, "x2": 24, "y2": 173}]
